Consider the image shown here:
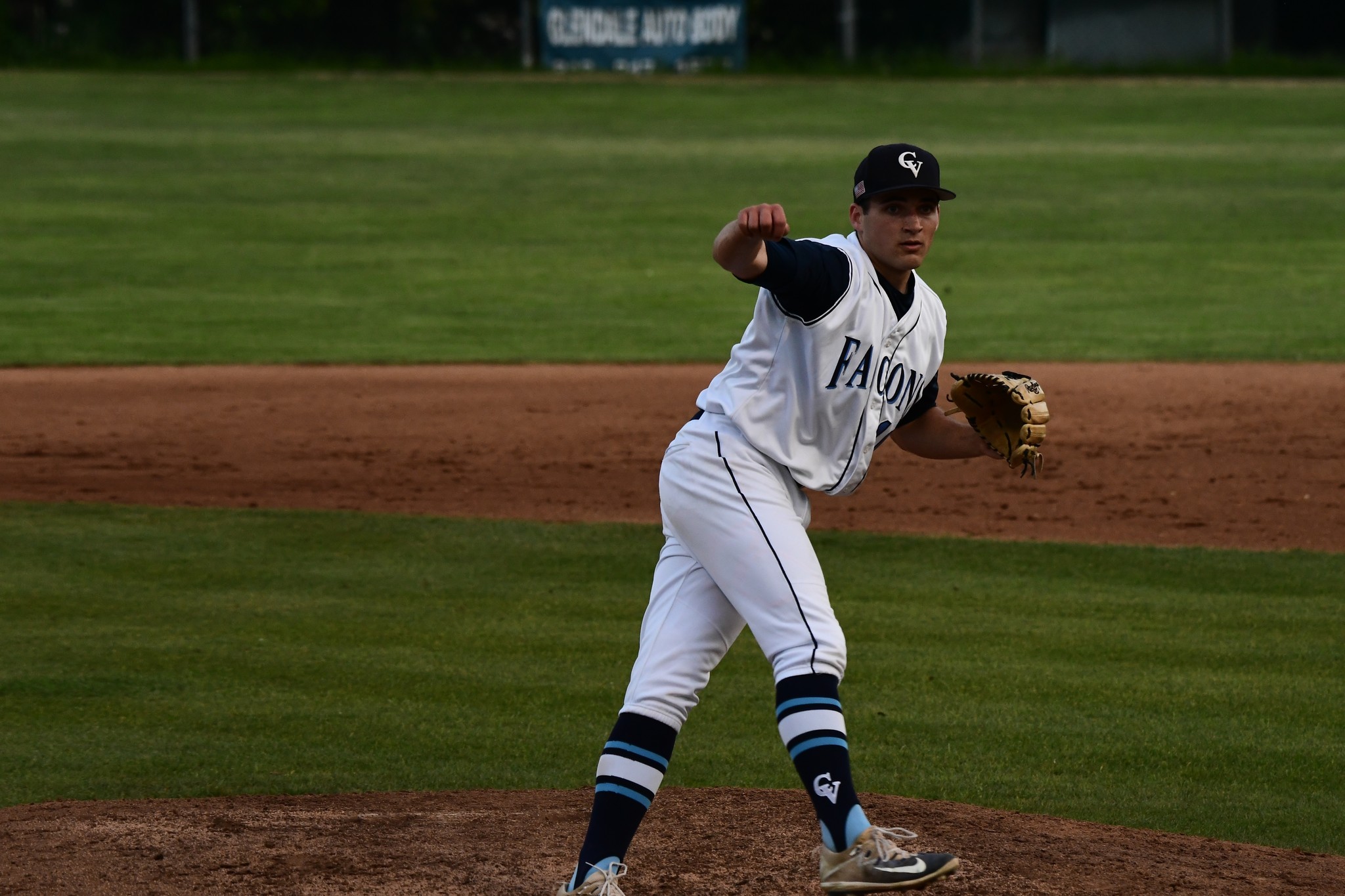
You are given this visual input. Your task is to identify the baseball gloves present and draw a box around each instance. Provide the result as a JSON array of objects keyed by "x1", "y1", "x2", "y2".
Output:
[{"x1": 950, "y1": 370, "x2": 1050, "y2": 469}]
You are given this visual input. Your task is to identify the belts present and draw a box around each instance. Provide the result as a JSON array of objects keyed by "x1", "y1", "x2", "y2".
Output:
[{"x1": 689, "y1": 409, "x2": 705, "y2": 422}]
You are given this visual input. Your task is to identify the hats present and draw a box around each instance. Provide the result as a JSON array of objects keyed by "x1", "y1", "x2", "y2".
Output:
[{"x1": 853, "y1": 143, "x2": 957, "y2": 203}]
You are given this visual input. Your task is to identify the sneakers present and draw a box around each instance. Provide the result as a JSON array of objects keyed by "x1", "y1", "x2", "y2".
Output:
[
  {"x1": 817, "y1": 825, "x2": 962, "y2": 893},
  {"x1": 556, "y1": 861, "x2": 628, "y2": 896}
]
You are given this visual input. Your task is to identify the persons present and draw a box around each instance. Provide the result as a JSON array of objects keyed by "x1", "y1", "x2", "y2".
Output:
[{"x1": 556, "y1": 142, "x2": 1051, "y2": 896}]
